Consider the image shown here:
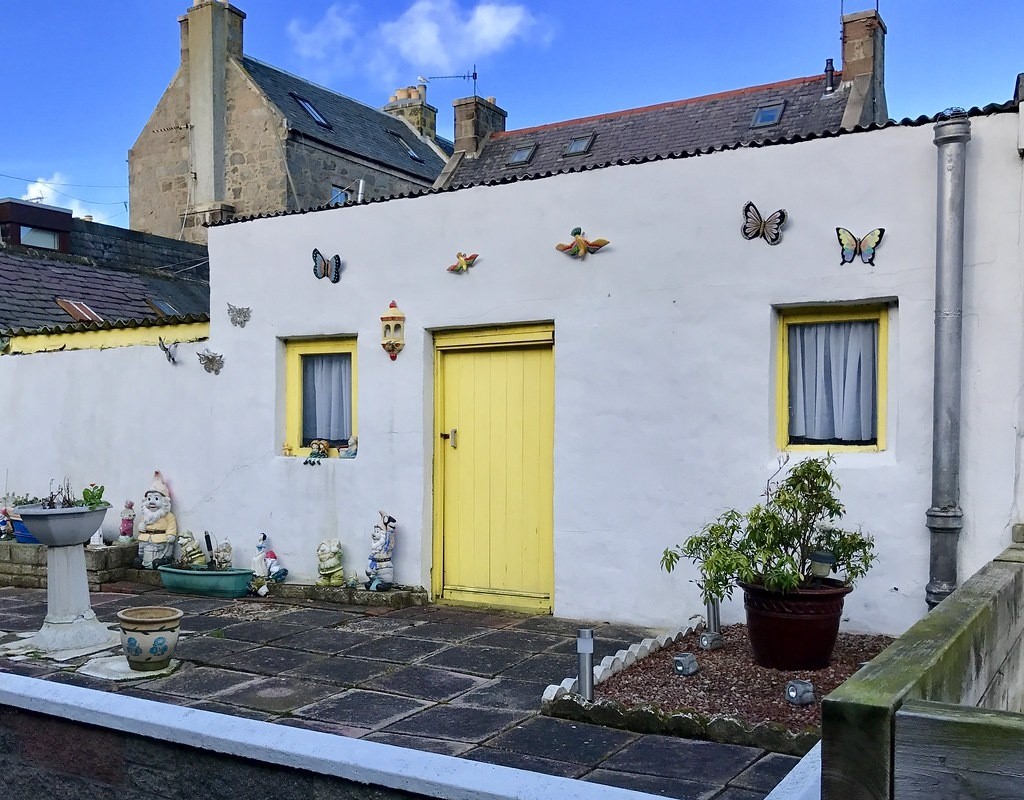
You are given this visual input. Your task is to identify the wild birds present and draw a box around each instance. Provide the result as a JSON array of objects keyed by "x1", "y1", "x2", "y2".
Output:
[
  {"x1": 555, "y1": 227, "x2": 610, "y2": 259},
  {"x1": 446, "y1": 252, "x2": 479, "y2": 273}
]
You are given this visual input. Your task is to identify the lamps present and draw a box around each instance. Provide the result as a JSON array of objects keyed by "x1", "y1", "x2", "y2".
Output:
[
  {"x1": 699, "y1": 632, "x2": 720, "y2": 651},
  {"x1": 784, "y1": 681, "x2": 814, "y2": 705},
  {"x1": 808, "y1": 547, "x2": 836, "y2": 579},
  {"x1": 672, "y1": 654, "x2": 697, "y2": 676}
]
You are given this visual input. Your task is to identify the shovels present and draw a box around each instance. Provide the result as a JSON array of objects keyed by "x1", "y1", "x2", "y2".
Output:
[{"x1": 205, "y1": 530, "x2": 217, "y2": 569}]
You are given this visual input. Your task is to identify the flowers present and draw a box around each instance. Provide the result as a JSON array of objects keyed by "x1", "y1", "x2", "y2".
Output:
[{"x1": 42, "y1": 477, "x2": 110, "y2": 510}]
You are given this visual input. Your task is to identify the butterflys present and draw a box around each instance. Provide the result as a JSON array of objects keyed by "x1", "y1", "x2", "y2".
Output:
[
  {"x1": 312, "y1": 248, "x2": 341, "y2": 283},
  {"x1": 740, "y1": 201, "x2": 787, "y2": 245},
  {"x1": 159, "y1": 336, "x2": 179, "y2": 363},
  {"x1": 836, "y1": 227, "x2": 885, "y2": 266}
]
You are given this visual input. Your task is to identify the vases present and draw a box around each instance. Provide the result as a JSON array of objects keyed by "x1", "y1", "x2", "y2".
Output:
[
  {"x1": 11, "y1": 516, "x2": 42, "y2": 544},
  {"x1": 118, "y1": 605, "x2": 183, "y2": 671},
  {"x1": 13, "y1": 502, "x2": 113, "y2": 545},
  {"x1": 157, "y1": 565, "x2": 255, "y2": 598}
]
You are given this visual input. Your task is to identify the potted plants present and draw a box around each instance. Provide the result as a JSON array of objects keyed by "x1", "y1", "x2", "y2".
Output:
[{"x1": 660, "y1": 453, "x2": 882, "y2": 671}]
